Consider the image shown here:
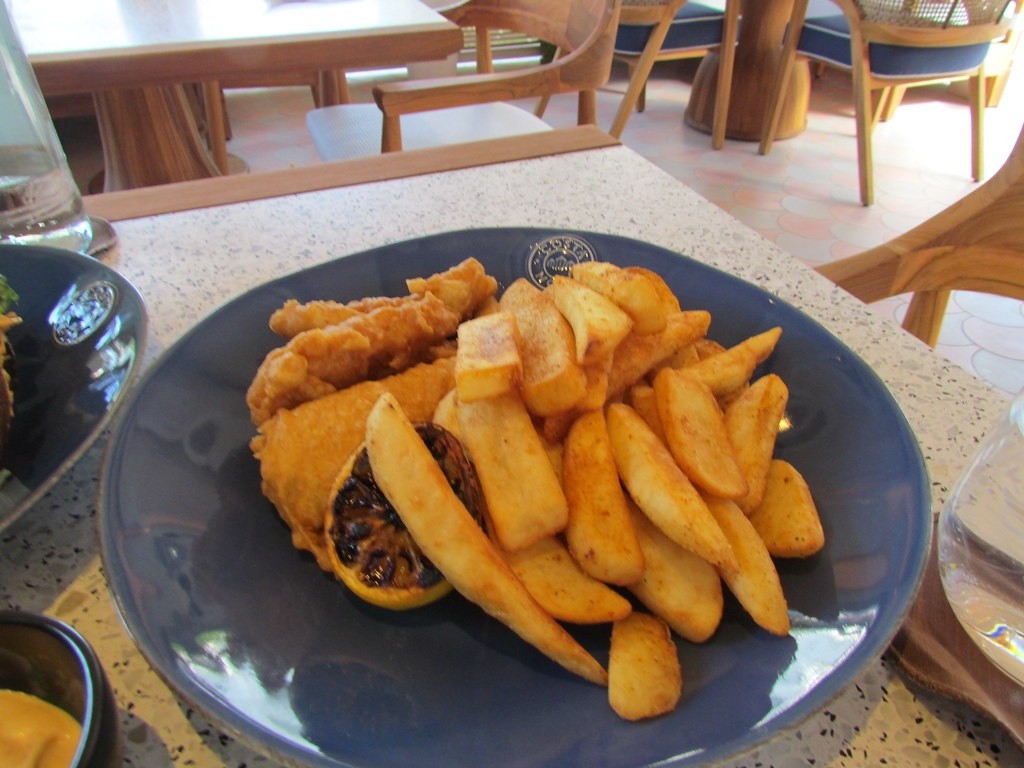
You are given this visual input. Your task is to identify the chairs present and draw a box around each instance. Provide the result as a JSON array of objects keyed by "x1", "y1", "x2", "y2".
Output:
[
  {"x1": 814, "y1": 122, "x2": 1024, "y2": 349},
  {"x1": 756, "y1": 0, "x2": 1016, "y2": 210},
  {"x1": 306, "y1": 0, "x2": 620, "y2": 159},
  {"x1": 533, "y1": 0, "x2": 743, "y2": 150}
]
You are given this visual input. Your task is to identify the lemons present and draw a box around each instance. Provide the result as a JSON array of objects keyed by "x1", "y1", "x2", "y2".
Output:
[{"x1": 324, "y1": 421, "x2": 485, "y2": 611}]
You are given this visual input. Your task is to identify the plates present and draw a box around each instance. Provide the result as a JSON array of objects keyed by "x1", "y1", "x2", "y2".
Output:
[
  {"x1": 0, "y1": 243, "x2": 149, "y2": 526},
  {"x1": 96, "y1": 226, "x2": 933, "y2": 768}
]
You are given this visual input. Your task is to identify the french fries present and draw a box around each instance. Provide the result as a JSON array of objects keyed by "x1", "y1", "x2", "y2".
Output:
[{"x1": 368, "y1": 261, "x2": 824, "y2": 723}]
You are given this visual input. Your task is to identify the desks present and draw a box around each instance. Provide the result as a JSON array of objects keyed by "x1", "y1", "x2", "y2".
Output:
[
  {"x1": 0, "y1": 125, "x2": 1024, "y2": 768},
  {"x1": 2, "y1": 0, "x2": 465, "y2": 197}
]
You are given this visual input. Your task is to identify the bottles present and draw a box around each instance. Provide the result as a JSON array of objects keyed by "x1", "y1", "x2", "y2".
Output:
[
  {"x1": 937, "y1": 385, "x2": 1024, "y2": 687},
  {"x1": 1, "y1": 0, "x2": 93, "y2": 255}
]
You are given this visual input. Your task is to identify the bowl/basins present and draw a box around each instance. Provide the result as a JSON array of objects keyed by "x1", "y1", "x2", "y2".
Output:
[{"x1": 0, "y1": 610, "x2": 119, "y2": 768}]
